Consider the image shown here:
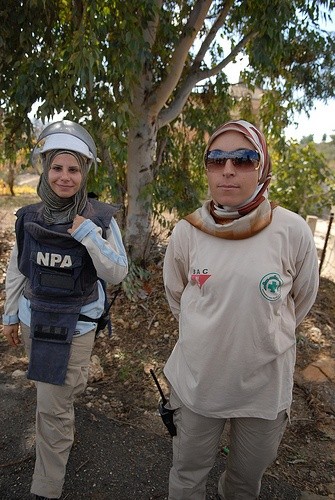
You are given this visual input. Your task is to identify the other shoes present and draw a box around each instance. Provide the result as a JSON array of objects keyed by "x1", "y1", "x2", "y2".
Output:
[{"x1": 30, "y1": 438, "x2": 36, "y2": 459}]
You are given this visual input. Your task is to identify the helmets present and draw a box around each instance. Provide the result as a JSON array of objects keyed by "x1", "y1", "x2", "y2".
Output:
[{"x1": 28, "y1": 120, "x2": 97, "y2": 180}]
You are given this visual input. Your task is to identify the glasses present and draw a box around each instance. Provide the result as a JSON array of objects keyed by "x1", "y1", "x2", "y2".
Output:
[{"x1": 204, "y1": 149, "x2": 260, "y2": 172}]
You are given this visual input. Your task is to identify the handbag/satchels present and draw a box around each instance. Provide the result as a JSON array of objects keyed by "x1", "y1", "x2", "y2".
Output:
[{"x1": 99, "y1": 278, "x2": 109, "y2": 329}]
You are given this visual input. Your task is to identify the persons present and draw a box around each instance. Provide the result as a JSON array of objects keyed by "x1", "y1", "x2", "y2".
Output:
[
  {"x1": 163, "y1": 120, "x2": 319, "y2": 500},
  {"x1": 3, "y1": 120, "x2": 128, "y2": 500}
]
